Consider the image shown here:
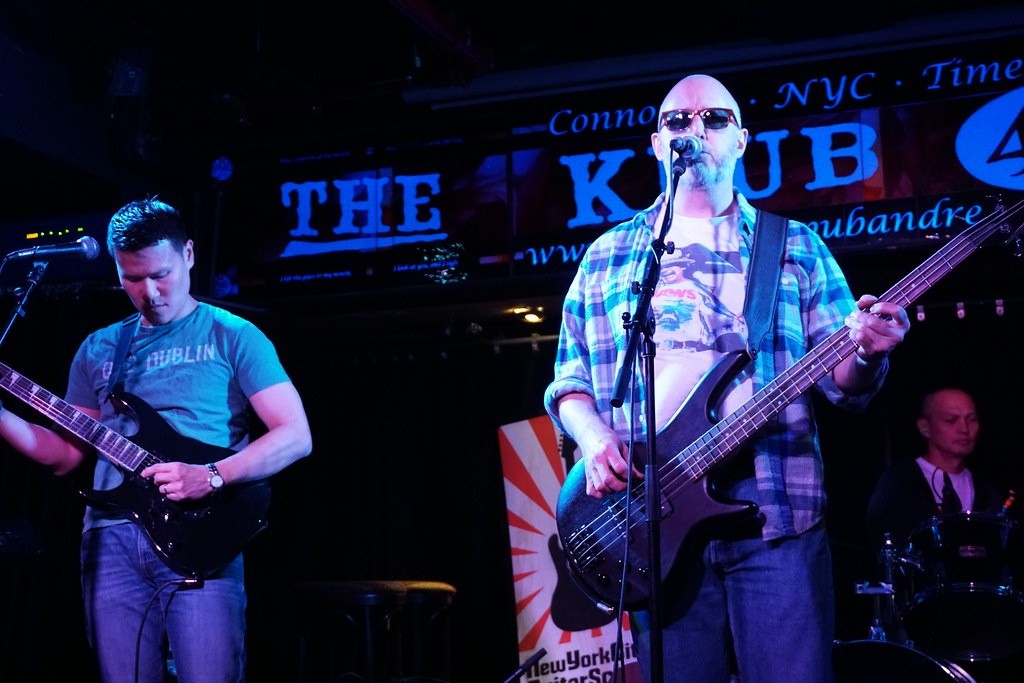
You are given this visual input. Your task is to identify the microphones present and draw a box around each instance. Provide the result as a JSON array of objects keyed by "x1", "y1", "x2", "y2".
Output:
[
  {"x1": 4, "y1": 236, "x2": 101, "y2": 260},
  {"x1": 669, "y1": 135, "x2": 703, "y2": 162}
]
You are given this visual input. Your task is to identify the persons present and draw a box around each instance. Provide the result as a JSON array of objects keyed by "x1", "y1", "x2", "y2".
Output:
[
  {"x1": 0, "y1": 196, "x2": 314, "y2": 683},
  {"x1": 892, "y1": 388, "x2": 1007, "y2": 543},
  {"x1": 544, "y1": 75, "x2": 909, "y2": 683}
]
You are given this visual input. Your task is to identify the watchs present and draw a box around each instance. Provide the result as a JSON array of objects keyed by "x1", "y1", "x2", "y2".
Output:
[{"x1": 205, "y1": 461, "x2": 225, "y2": 492}]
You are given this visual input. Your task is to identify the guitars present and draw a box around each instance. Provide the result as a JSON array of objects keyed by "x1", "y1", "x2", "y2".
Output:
[
  {"x1": 556, "y1": 201, "x2": 1024, "y2": 609},
  {"x1": 0, "y1": 362, "x2": 274, "y2": 579}
]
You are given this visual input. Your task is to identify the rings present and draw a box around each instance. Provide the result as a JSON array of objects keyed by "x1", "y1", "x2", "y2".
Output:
[{"x1": 164, "y1": 484, "x2": 168, "y2": 493}]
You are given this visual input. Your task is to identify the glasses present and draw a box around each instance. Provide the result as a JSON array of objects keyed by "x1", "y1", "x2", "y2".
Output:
[{"x1": 652, "y1": 108, "x2": 744, "y2": 137}]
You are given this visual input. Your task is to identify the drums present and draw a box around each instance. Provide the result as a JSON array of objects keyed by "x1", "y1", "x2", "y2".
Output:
[
  {"x1": 879, "y1": 507, "x2": 1024, "y2": 681},
  {"x1": 828, "y1": 637, "x2": 972, "y2": 683}
]
